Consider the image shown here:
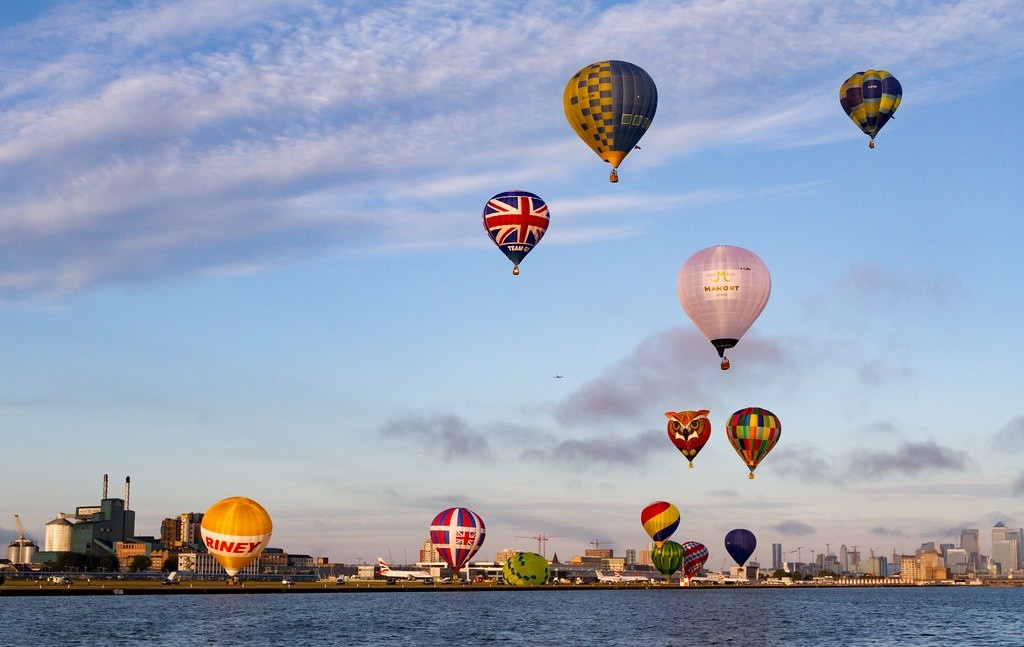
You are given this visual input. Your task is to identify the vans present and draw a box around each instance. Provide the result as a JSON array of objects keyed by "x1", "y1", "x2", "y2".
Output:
[{"x1": 562, "y1": 577, "x2": 585, "y2": 586}]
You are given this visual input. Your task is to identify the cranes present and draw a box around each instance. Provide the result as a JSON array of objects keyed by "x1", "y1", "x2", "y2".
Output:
[{"x1": 513, "y1": 533, "x2": 617, "y2": 560}]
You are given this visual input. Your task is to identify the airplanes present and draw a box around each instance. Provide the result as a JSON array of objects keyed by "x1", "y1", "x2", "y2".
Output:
[
  {"x1": 375, "y1": 557, "x2": 433, "y2": 584},
  {"x1": 718, "y1": 572, "x2": 750, "y2": 584},
  {"x1": 596, "y1": 569, "x2": 650, "y2": 584}
]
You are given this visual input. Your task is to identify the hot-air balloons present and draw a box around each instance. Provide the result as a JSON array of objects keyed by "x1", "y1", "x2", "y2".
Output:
[
  {"x1": 650, "y1": 540, "x2": 685, "y2": 585},
  {"x1": 726, "y1": 407, "x2": 781, "y2": 479},
  {"x1": 839, "y1": 69, "x2": 903, "y2": 148},
  {"x1": 724, "y1": 528, "x2": 756, "y2": 572},
  {"x1": 563, "y1": 60, "x2": 658, "y2": 183},
  {"x1": 677, "y1": 244, "x2": 771, "y2": 371},
  {"x1": 499, "y1": 552, "x2": 550, "y2": 585},
  {"x1": 680, "y1": 541, "x2": 709, "y2": 586},
  {"x1": 482, "y1": 189, "x2": 550, "y2": 275},
  {"x1": 665, "y1": 409, "x2": 711, "y2": 468},
  {"x1": 201, "y1": 497, "x2": 272, "y2": 585},
  {"x1": 640, "y1": 500, "x2": 680, "y2": 553},
  {"x1": 430, "y1": 507, "x2": 485, "y2": 580}
]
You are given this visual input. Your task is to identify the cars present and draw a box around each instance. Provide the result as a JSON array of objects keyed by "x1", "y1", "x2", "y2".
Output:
[
  {"x1": 281, "y1": 578, "x2": 294, "y2": 586},
  {"x1": 59, "y1": 577, "x2": 74, "y2": 585},
  {"x1": 53, "y1": 575, "x2": 64, "y2": 585},
  {"x1": 336, "y1": 579, "x2": 346, "y2": 586},
  {"x1": 163, "y1": 579, "x2": 181, "y2": 585},
  {"x1": 498, "y1": 579, "x2": 504, "y2": 585}
]
